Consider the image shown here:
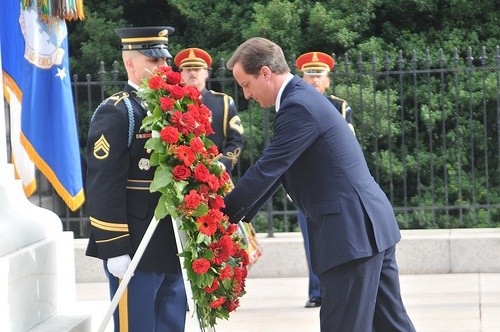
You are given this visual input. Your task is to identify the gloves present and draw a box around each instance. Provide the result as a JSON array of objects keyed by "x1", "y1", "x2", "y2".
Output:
[{"x1": 107, "y1": 254, "x2": 135, "y2": 279}]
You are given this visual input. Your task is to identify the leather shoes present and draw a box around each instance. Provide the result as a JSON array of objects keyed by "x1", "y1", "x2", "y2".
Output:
[{"x1": 304, "y1": 296, "x2": 322, "y2": 307}]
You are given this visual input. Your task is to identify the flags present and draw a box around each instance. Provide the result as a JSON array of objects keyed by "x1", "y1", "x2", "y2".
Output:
[{"x1": 0, "y1": 0, "x2": 85, "y2": 212}]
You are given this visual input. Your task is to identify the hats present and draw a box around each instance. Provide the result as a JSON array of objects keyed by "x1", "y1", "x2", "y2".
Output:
[
  {"x1": 295, "y1": 51, "x2": 336, "y2": 76},
  {"x1": 174, "y1": 47, "x2": 212, "y2": 71},
  {"x1": 114, "y1": 25, "x2": 173, "y2": 59}
]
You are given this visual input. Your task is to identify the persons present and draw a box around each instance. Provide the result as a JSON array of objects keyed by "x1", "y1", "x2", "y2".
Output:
[
  {"x1": 292, "y1": 51, "x2": 357, "y2": 308},
  {"x1": 173, "y1": 47, "x2": 246, "y2": 176},
  {"x1": 82, "y1": 26, "x2": 187, "y2": 332},
  {"x1": 218, "y1": 37, "x2": 416, "y2": 332}
]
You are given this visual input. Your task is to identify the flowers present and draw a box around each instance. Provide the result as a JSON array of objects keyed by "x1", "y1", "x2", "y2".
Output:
[{"x1": 130, "y1": 64, "x2": 250, "y2": 332}]
